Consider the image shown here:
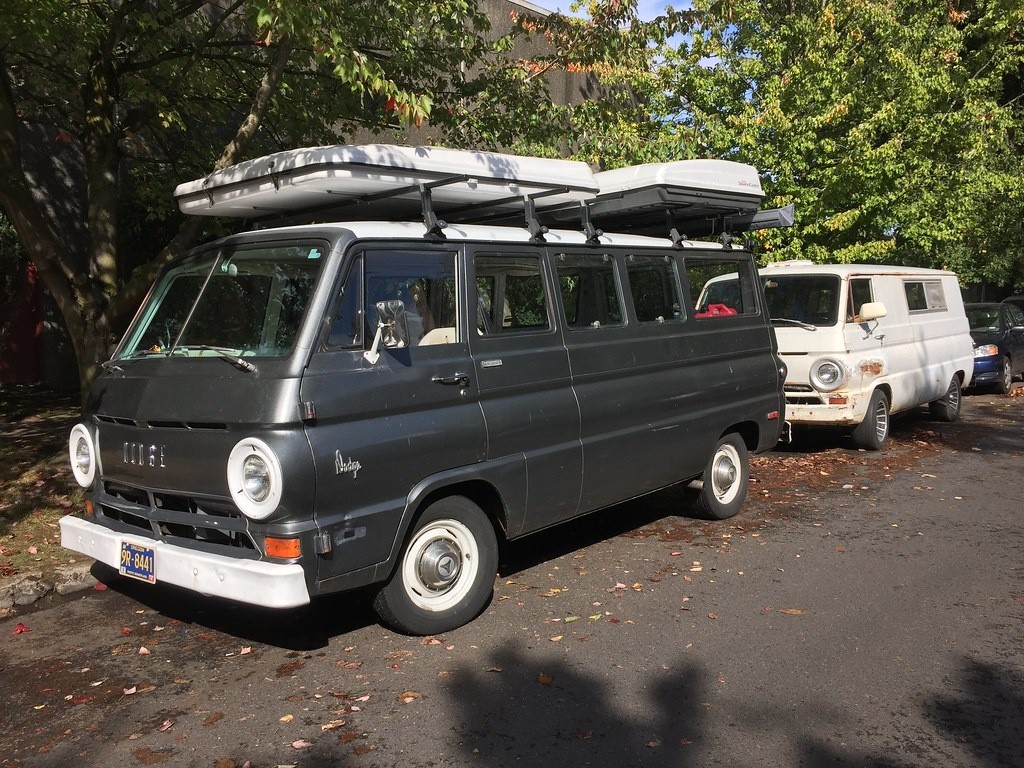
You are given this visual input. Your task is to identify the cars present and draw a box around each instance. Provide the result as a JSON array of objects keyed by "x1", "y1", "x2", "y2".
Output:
[{"x1": 947, "y1": 300, "x2": 1024, "y2": 395}]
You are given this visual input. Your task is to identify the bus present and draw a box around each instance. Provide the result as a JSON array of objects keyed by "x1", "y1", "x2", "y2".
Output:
[
  {"x1": 686, "y1": 253, "x2": 976, "y2": 449},
  {"x1": 59, "y1": 143, "x2": 791, "y2": 640}
]
